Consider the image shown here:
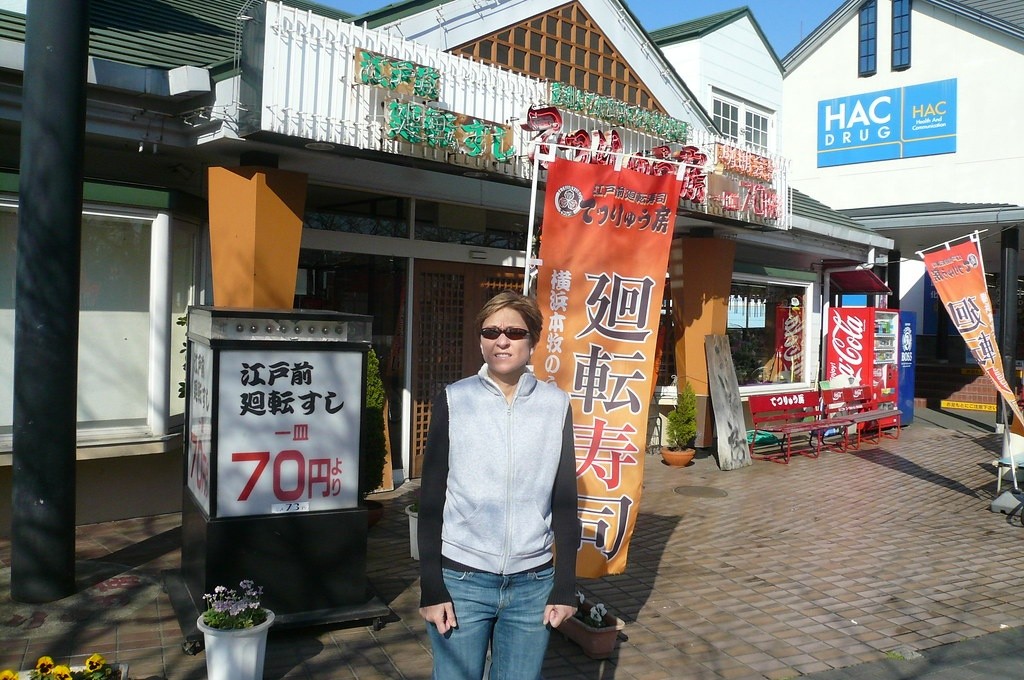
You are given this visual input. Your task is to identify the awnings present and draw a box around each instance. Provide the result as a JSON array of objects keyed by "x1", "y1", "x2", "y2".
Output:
[{"x1": 821, "y1": 259, "x2": 893, "y2": 295}]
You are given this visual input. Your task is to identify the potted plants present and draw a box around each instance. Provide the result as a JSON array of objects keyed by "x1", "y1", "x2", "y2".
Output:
[{"x1": 662, "y1": 381, "x2": 699, "y2": 470}]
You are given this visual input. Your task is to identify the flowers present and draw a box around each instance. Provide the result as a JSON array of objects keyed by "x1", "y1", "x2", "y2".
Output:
[
  {"x1": 202, "y1": 580, "x2": 265, "y2": 631},
  {"x1": 0, "y1": 656, "x2": 101, "y2": 680}
]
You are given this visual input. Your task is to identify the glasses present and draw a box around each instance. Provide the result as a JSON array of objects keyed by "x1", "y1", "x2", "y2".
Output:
[{"x1": 480, "y1": 327, "x2": 530, "y2": 340}]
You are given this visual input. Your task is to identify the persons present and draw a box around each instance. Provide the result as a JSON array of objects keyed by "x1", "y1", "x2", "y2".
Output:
[{"x1": 417, "y1": 290, "x2": 578, "y2": 680}]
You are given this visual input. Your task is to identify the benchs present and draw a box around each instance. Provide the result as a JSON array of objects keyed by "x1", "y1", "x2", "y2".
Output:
[
  {"x1": 822, "y1": 385, "x2": 903, "y2": 449},
  {"x1": 749, "y1": 390, "x2": 853, "y2": 464}
]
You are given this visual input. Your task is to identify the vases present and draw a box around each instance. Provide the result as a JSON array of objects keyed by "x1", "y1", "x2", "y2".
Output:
[
  {"x1": 16, "y1": 663, "x2": 130, "y2": 680},
  {"x1": 197, "y1": 608, "x2": 276, "y2": 680}
]
